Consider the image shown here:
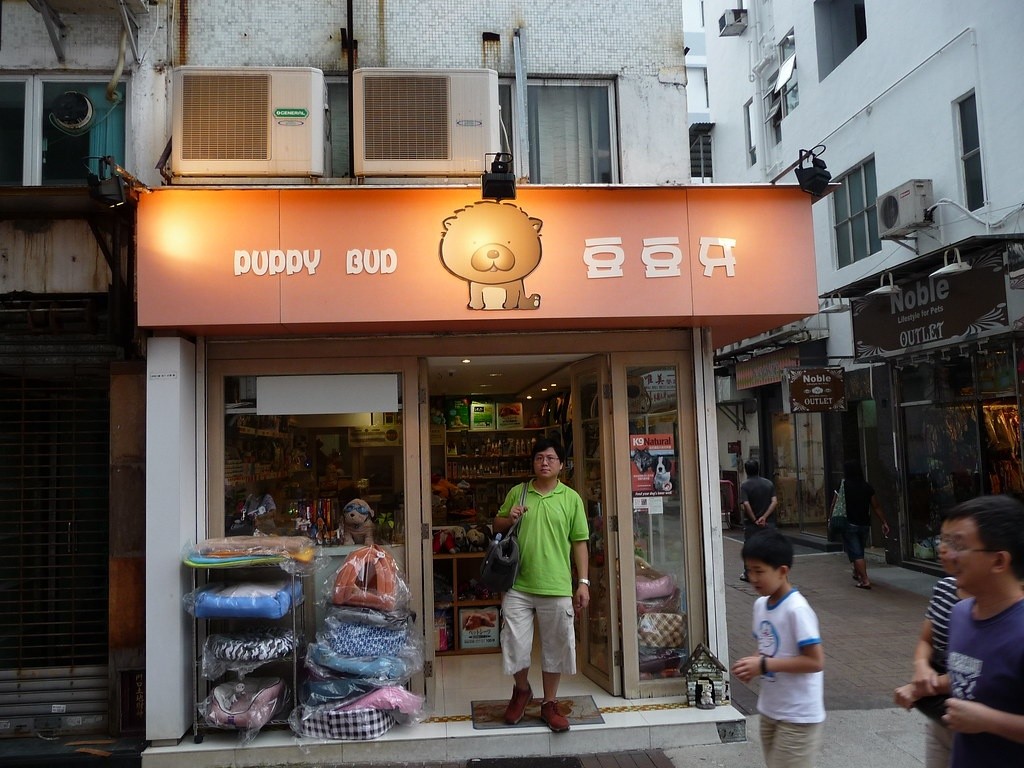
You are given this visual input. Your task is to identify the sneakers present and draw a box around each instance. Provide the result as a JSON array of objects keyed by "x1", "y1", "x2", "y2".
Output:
[
  {"x1": 503, "y1": 681, "x2": 533, "y2": 725},
  {"x1": 541, "y1": 700, "x2": 570, "y2": 733}
]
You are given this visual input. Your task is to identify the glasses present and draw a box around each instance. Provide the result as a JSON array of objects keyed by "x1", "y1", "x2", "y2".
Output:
[
  {"x1": 951, "y1": 540, "x2": 996, "y2": 556},
  {"x1": 534, "y1": 455, "x2": 560, "y2": 463},
  {"x1": 935, "y1": 535, "x2": 953, "y2": 547}
]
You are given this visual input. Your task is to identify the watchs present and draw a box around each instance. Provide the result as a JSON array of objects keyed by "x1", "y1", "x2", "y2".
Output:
[{"x1": 578, "y1": 578, "x2": 590, "y2": 586}]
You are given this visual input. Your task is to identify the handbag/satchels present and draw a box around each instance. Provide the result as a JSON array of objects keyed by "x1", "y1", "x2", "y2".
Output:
[
  {"x1": 478, "y1": 482, "x2": 529, "y2": 594},
  {"x1": 830, "y1": 479, "x2": 847, "y2": 521}
]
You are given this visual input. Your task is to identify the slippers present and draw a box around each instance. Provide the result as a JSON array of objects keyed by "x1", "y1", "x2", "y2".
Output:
[
  {"x1": 852, "y1": 573, "x2": 862, "y2": 581},
  {"x1": 855, "y1": 582, "x2": 872, "y2": 590}
]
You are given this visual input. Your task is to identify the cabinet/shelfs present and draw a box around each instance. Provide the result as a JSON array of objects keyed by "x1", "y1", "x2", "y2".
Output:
[{"x1": 222, "y1": 419, "x2": 606, "y2": 657}]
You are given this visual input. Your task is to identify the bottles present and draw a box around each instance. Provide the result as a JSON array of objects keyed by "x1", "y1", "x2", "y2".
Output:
[
  {"x1": 447, "y1": 431, "x2": 538, "y2": 521},
  {"x1": 540, "y1": 388, "x2": 598, "y2": 426},
  {"x1": 288, "y1": 496, "x2": 405, "y2": 547}
]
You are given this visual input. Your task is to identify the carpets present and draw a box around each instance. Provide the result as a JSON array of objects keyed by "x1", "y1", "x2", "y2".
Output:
[{"x1": 471, "y1": 695, "x2": 605, "y2": 730}]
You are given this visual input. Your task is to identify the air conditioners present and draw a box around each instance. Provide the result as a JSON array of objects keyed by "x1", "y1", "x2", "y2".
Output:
[
  {"x1": 353, "y1": 67, "x2": 500, "y2": 178},
  {"x1": 717, "y1": 8, "x2": 749, "y2": 37},
  {"x1": 876, "y1": 178, "x2": 936, "y2": 239},
  {"x1": 171, "y1": 65, "x2": 326, "y2": 177}
]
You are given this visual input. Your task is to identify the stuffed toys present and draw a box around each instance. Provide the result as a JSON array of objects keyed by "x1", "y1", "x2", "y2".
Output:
[
  {"x1": 344, "y1": 500, "x2": 382, "y2": 547},
  {"x1": 433, "y1": 524, "x2": 492, "y2": 554}
]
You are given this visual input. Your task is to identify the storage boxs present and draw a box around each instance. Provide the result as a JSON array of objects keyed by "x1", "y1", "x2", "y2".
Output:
[
  {"x1": 469, "y1": 404, "x2": 496, "y2": 430},
  {"x1": 495, "y1": 402, "x2": 524, "y2": 429}
]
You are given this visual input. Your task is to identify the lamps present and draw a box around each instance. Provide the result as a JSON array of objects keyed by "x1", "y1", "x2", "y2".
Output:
[
  {"x1": 795, "y1": 157, "x2": 831, "y2": 197},
  {"x1": 819, "y1": 304, "x2": 849, "y2": 313},
  {"x1": 929, "y1": 263, "x2": 972, "y2": 277},
  {"x1": 97, "y1": 175, "x2": 126, "y2": 208},
  {"x1": 481, "y1": 153, "x2": 515, "y2": 201},
  {"x1": 865, "y1": 285, "x2": 902, "y2": 296}
]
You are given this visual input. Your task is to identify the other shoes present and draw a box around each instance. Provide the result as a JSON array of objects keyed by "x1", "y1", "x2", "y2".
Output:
[{"x1": 738, "y1": 572, "x2": 749, "y2": 583}]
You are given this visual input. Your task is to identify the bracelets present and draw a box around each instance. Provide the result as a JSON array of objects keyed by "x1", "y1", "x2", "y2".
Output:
[
  {"x1": 828, "y1": 519, "x2": 831, "y2": 521},
  {"x1": 760, "y1": 656, "x2": 768, "y2": 675}
]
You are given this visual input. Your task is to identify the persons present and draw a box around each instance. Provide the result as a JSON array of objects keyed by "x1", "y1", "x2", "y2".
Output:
[
  {"x1": 732, "y1": 531, "x2": 827, "y2": 768},
  {"x1": 894, "y1": 514, "x2": 972, "y2": 768},
  {"x1": 242, "y1": 481, "x2": 277, "y2": 530},
  {"x1": 740, "y1": 460, "x2": 779, "y2": 582},
  {"x1": 942, "y1": 496, "x2": 1024, "y2": 768},
  {"x1": 828, "y1": 461, "x2": 889, "y2": 590},
  {"x1": 494, "y1": 440, "x2": 590, "y2": 731},
  {"x1": 432, "y1": 466, "x2": 465, "y2": 506}
]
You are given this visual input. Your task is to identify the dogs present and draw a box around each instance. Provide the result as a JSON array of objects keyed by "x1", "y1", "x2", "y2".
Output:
[
  {"x1": 651, "y1": 455, "x2": 672, "y2": 491},
  {"x1": 342, "y1": 498, "x2": 375, "y2": 546}
]
